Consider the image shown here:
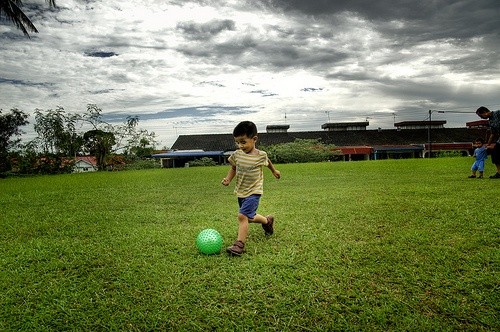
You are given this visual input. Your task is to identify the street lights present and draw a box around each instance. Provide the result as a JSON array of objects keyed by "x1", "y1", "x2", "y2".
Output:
[{"x1": 429, "y1": 109, "x2": 445, "y2": 158}]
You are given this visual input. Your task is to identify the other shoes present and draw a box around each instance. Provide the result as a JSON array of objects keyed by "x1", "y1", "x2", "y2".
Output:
[
  {"x1": 468, "y1": 175, "x2": 476, "y2": 178},
  {"x1": 477, "y1": 175, "x2": 483, "y2": 178},
  {"x1": 489, "y1": 172, "x2": 500, "y2": 179}
]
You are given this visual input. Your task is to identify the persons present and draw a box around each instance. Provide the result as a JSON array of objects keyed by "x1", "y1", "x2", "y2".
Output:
[
  {"x1": 221, "y1": 121, "x2": 281, "y2": 257},
  {"x1": 467, "y1": 136, "x2": 488, "y2": 179},
  {"x1": 476, "y1": 106, "x2": 500, "y2": 178}
]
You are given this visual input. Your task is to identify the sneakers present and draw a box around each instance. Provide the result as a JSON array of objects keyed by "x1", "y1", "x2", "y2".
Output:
[
  {"x1": 227, "y1": 241, "x2": 246, "y2": 256},
  {"x1": 262, "y1": 215, "x2": 274, "y2": 236}
]
